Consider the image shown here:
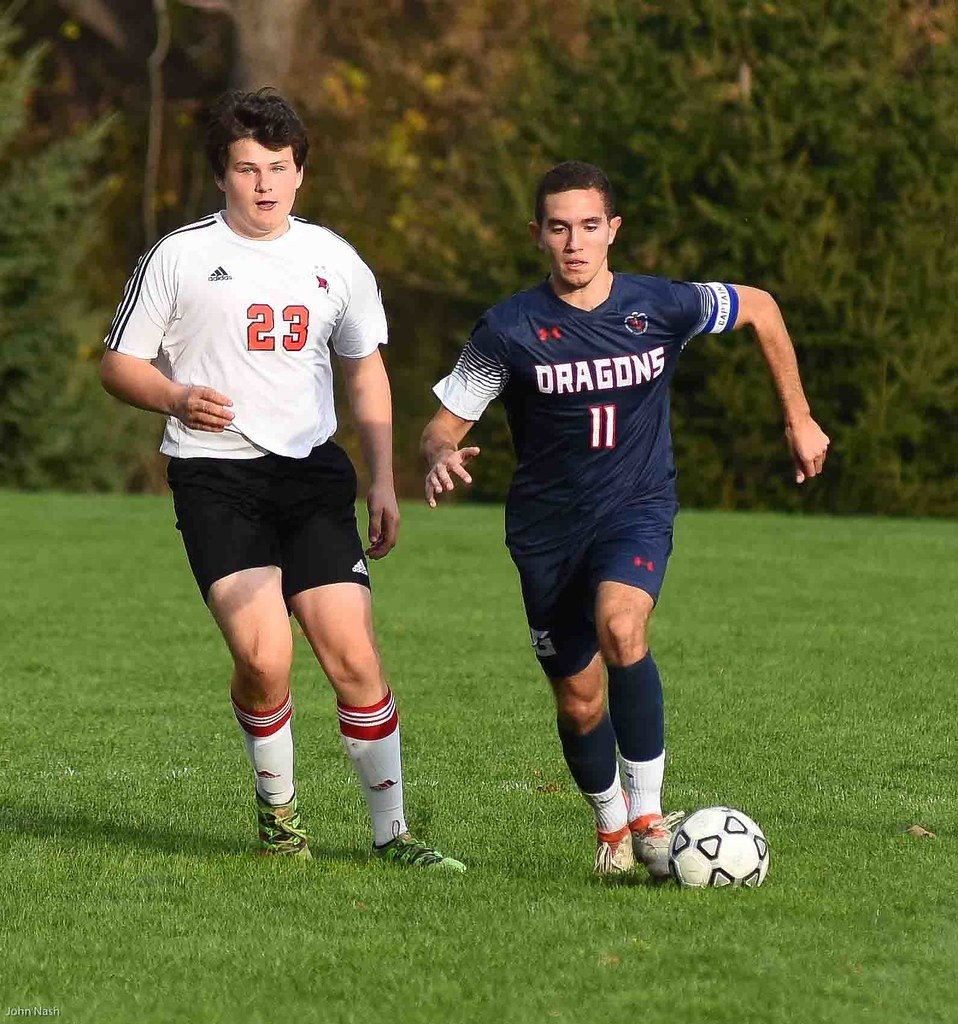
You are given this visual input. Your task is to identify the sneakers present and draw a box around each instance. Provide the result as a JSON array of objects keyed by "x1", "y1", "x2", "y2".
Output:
[
  {"x1": 250, "y1": 787, "x2": 313, "y2": 861},
  {"x1": 592, "y1": 788, "x2": 637, "y2": 879},
  {"x1": 366, "y1": 819, "x2": 472, "y2": 877},
  {"x1": 625, "y1": 808, "x2": 686, "y2": 878}
]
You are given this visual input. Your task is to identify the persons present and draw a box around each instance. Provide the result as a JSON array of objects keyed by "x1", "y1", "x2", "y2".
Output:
[
  {"x1": 420, "y1": 161, "x2": 830, "y2": 881},
  {"x1": 99, "y1": 89, "x2": 467, "y2": 872}
]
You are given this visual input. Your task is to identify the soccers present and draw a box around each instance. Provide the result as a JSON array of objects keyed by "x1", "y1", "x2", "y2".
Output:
[{"x1": 667, "y1": 803, "x2": 770, "y2": 888}]
